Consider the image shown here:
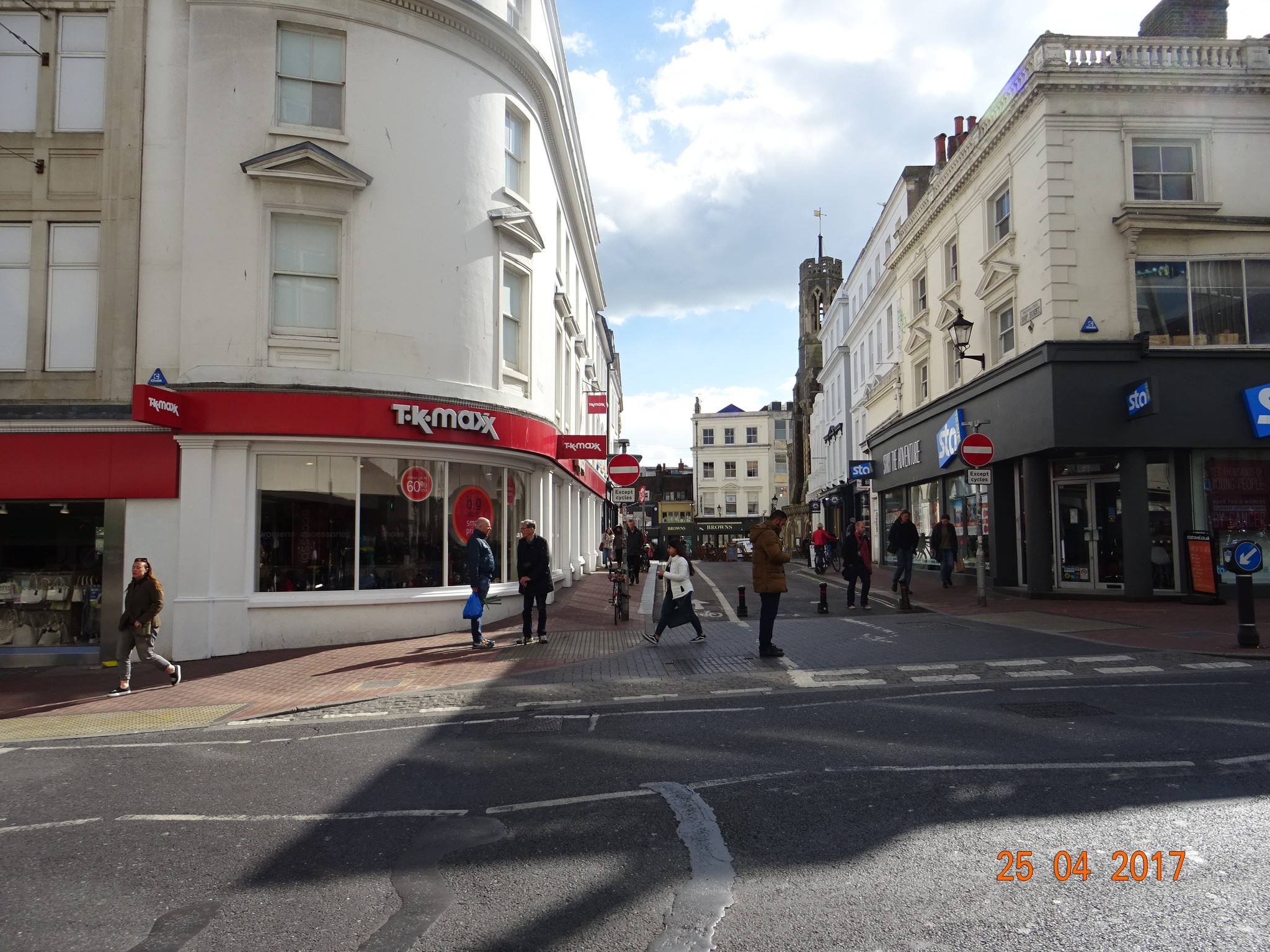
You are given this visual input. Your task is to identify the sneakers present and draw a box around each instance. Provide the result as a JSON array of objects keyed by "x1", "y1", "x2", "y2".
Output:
[
  {"x1": 690, "y1": 635, "x2": 707, "y2": 643},
  {"x1": 516, "y1": 635, "x2": 534, "y2": 643},
  {"x1": 538, "y1": 635, "x2": 548, "y2": 643},
  {"x1": 643, "y1": 633, "x2": 659, "y2": 645},
  {"x1": 473, "y1": 638, "x2": 495, "y2": 649}
]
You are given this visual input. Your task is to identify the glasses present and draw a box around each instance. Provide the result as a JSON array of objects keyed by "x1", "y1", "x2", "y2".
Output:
[
  {"x1": 135, "y1": 558, "x2": 148, "y2": 561},
  {"x1": 521, "y1": 527, "x2": 532, "y2": 531}
]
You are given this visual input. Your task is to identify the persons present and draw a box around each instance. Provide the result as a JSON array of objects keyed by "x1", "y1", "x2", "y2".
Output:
[
  {"x1": 749, "y1": 509, "x2": 792, "y2": 658},
  {"x1": 930, "y1": 514, "x2": 958, "y2": 588},
  {"x1": 811, "y1": 523, "x2": 836, "y2": 574},
  {"x1": 642, "y1": 539, "x2": 707, "y2": 645},
  {"x1": 846, "y1": 518, "x2": 855, "y2": 537},
  {"x1": 886, "y1": 509, "x2": 919, "y2": 594},
  {"x1": 802, "y1": 537, "x2": 811, "y2": 551},
  {"x1": 840, "y1": 521, "x2": 872, "y2": 610},
  {"x1": 516, "y1": 519, "x2": 554, "y2": 643},
  {"x1": 463, "y1": 517, "x2": 495, "y2": 649},
  {"x1": 107, "y1": 557, "x2": 181, "y2": 697},
  {"x1": 601, "y1": 519, "x2": 687, "y2": 586}
]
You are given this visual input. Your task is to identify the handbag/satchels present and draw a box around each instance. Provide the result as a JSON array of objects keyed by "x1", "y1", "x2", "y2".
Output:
[
  {"x1": 667, "y1": 608, "x2": 691, "y2": 629},
  {"x1": 462, "y1": 591, "x2": 483, "y2": 619},
  {"x1": 0, "y1": 575, "x2": 103, "y2": 646},
  {"x1": 954, "y1": 557, "x2": 966, "y2": 572},
  {"x1": 599, "y1": 542, "x2": 604, "y2": 551},
  {"x1": 841, "y1": 566, "x2": 850, "y2": 581}
]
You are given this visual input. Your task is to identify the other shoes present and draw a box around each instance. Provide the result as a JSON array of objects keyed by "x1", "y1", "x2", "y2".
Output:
[
  {"x1": 629, "y1": 583, "x2": 634, "y2": 586},
  {"x1": 771, "y1": 644, "x2": 783, "y2": 652},
  {"x1": 943, "y1": 581, "x2": 947, "y2": 588},
  {"x1": 863, "y1": 604, "x2": 871, "y2": 609},
  {"x1": 609, "y1": 562, "x2": 612, "y2": 568},
  {"x1": 849, "y1": 605, "x2": 855, "y2": 609},
  {"x1": 107, "y1": 686, "x2": 130, "y2": 697},
  {"x1": 759, "y1": 649, "x2": 785, "y2": 658},
  {"x1": 947, "y1": 578, "x2": 953, "y2": 586},
  {"x1": 907, "y1": 590, "x2": 913, "y2": 595},
  {"x1": 892, "y1": 582, "x2": 898, "y2": 592},
  {"x1": 169, "y1": 664, "x2": 181, "y2": 687},
  {"x1": 605, "y1": 565, "x2": 608, "y2": 568},
  {"x1": 636, "y1": 577, "x2": 639, "y2": 584},
  {"x1": 618, "y1": 565, "x2": 621, "y2": 568}
]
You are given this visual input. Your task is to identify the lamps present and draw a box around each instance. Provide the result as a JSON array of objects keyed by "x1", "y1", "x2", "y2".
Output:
[{"x1": 947, "y1": 308, "x2": 985, "y2": 370}]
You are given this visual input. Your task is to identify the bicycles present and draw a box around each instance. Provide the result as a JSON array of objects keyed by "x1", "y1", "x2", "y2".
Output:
[
  {"x1": 814, "y1": 539, "x2": 841, "y2": 575},
  {"x1": 912, "y1": 536, "x2": 935, "y2": 569},
  {"x1": 639, "y1": 542, "x2": 652, "y2": 572},
  {"x1": 608, "y1": 564, "x2": 631, "y2": 626}
]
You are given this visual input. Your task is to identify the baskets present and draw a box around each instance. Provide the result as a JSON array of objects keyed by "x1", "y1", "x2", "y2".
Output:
[{"x1": 609, "y1": 569, "x2": 625, "y2": 582}]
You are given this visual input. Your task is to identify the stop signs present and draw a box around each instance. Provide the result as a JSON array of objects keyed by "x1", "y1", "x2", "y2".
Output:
[
  {"x1": 607, "y1": 453, "x2": 640, "y2": 486},
  {"x1": 961, "y1": 434, "x2": 995, "y2": 468}
]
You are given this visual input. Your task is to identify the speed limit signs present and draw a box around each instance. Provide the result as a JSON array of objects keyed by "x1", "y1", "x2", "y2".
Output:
[{"x1": 639, "y1": 487, "x2": 645, "y2": 503}]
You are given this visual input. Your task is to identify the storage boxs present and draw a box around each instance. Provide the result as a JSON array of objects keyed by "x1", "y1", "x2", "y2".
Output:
[
  {"x1": 1212, "y1": 333, "x2": 1239, "y2": 344},
  {"x1": 1149, "y1": 334, "x2": 1170, "y2": 346},
  {"x1": 1172, "y1": 334, "x2": 1207, "y2": 346}
]
